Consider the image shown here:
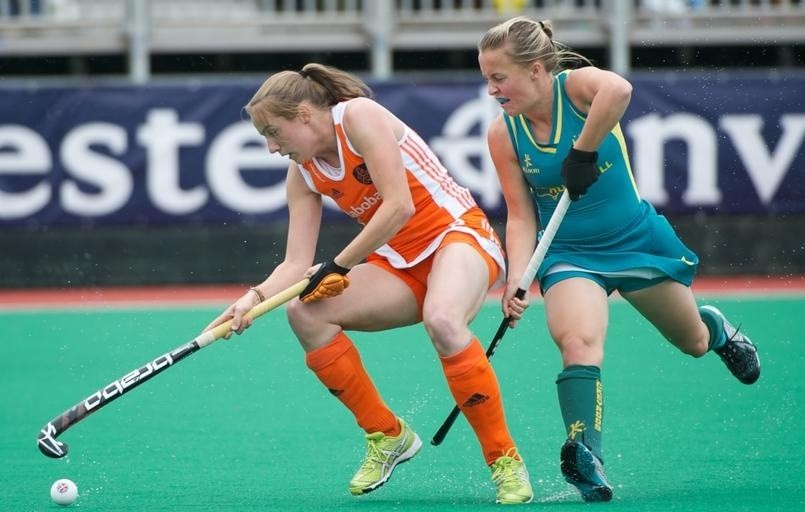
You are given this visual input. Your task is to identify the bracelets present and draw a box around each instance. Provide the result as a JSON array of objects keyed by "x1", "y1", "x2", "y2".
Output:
[{"x1": 250, "y1": 287, "x2": 267, "y2": 302}]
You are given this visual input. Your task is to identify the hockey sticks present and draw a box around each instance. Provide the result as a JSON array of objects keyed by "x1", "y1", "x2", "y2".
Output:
[
  {"x1": 431, "y1": 188, "x2": 572, "y2": 446},
  {"x1": 38, "y1": 278, "x2": 312, "y2": 457}
]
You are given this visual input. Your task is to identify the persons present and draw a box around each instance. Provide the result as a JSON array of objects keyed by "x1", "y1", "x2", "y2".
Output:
[
  {"x1": 478, "y1": 17, "x2": 761, "y2": 502},
  {"x1": 201, "y1": 63, "x2": 535, "y2": 505}
]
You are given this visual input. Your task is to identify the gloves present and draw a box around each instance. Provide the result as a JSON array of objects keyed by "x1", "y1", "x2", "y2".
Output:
[
  {"x1": 560, "y1": 146, "x2": 598, "y2": 202},
  {"x1": 299, "y1": 260, "x2": 351, "y2": 304}
]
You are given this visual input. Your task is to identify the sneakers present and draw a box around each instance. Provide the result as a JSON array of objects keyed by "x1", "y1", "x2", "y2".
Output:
[
  {"x1": 701, "y1": 305, "x2": 760, "y2": 384},
  {"x1": 490, "y1": 446, "x2": 534, "y2": 504},
  {"x1": 349, "y1": 417, "x2": 423, "y2": 496},
  {"x1": 560, "y1": 438, "x2": 613, "y2": 502}
]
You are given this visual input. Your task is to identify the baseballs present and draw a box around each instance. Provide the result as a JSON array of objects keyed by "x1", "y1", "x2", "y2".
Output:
[{"x1": 51, "y1": 478, "x2": 78, "y2": 506}]
